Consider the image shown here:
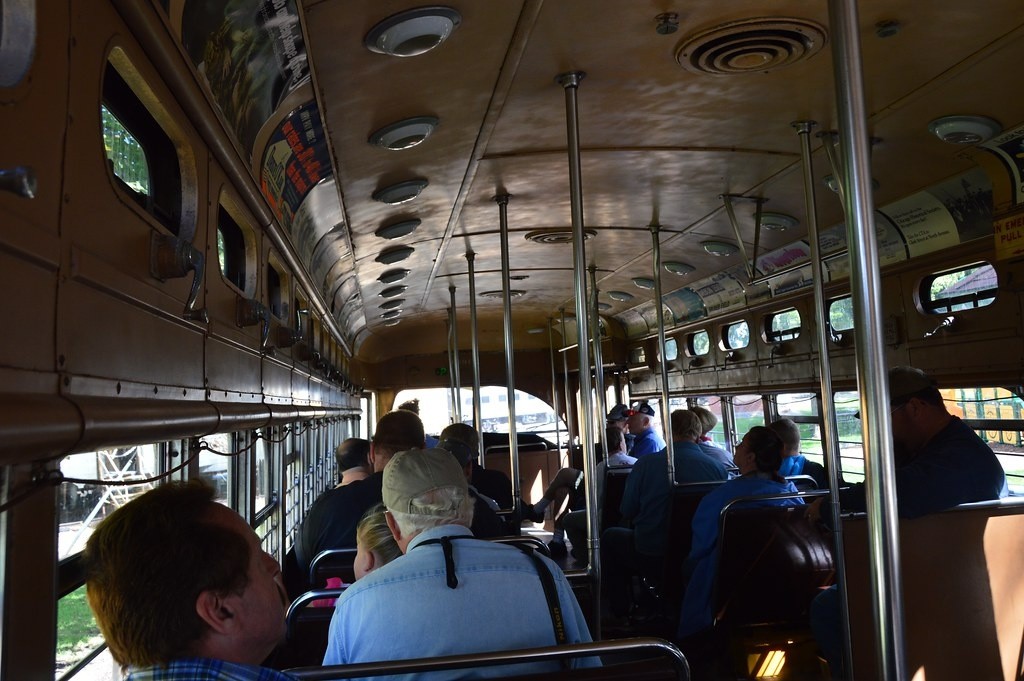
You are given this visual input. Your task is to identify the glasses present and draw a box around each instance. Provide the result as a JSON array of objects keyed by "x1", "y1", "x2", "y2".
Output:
[{"x1": 606, "y1": 420, "x2": 616, "y2": 424}]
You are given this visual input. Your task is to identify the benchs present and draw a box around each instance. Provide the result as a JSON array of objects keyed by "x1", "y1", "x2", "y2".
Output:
[{"x1": 270, "y1": 439, "x2": 1024, "y2": 681}]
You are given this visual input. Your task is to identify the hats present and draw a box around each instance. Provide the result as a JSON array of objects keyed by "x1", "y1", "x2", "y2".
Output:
[
  {"x1": 621, "y1": 400, "x2": 655, "y2": 417},
  {"x1": 371, "y1": 409, "x2": 424, "y2": 448},
  {"x1": 888, "y1": 365, "x2": 935, "y2": 399},
  {"x1": 438, "y1": 423, "x2": 478, "y2": 448},
  {"x1": 381, "y1": 447, "x2": 468, "y2": 515},
  {"x1": 606, "y1": 403, "x2": 627, "y2": 420},
  {"x1": 435, "y1": 438, "x2": 479, "y2": 467}
]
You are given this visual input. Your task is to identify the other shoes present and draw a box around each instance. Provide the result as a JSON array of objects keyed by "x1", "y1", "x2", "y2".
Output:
[
  {"x1": 521, "y1": 500, "x2": 545, "y2": 523},
  {"x1": 600, "y1": 612, "x2": 636, "y2": 633},
  {"x1": 547, "y1": 540, "x2": 567, "y2": 551}
]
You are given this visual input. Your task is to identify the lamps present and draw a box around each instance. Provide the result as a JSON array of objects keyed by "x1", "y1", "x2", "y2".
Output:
[
  {"x1": 375, "y1": 245, "x2": 415, "y2": 264},
  {"x1": 524, "y1": 211, "x2": 799, "y2": 334},
  {"x1": 821, "y1": 173, "x2": 881, "y2": 196},
  {"x1": 375, "y1": 220, "x2": 421, "y2": 238},
  {"x1": 928, "y1": 115, "x2": 1001, "y2": 145},
  {"x1": 373, "y1": 180, "x2": 429, "y2": 204},
  {"x1": 364, "y1": 6, "x2": 462, "y2": 57},
  {"x1": 366, "y1": 116, "x2": 439, "y2": 151},
  {"x1": 377, "y1": 268, "x2": 410, "y2": 283},
  {"x1": 377, "y1": 285, "x2": 405, "y2": 327}
]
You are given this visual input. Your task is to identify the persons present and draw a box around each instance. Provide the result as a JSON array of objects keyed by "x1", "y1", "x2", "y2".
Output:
[
  {"x1": 296, "y1": 365, "x2": 1011, "y2": 681},
  {"x1": 86, "y1": 480, "x2": 299, "y2": 681},
  {"x1": 59, "y1": 486, "x2": 103, "y2": 522},
  {"x1": 203, "y1": 472, "x2": 230, "y2": 498}
]
